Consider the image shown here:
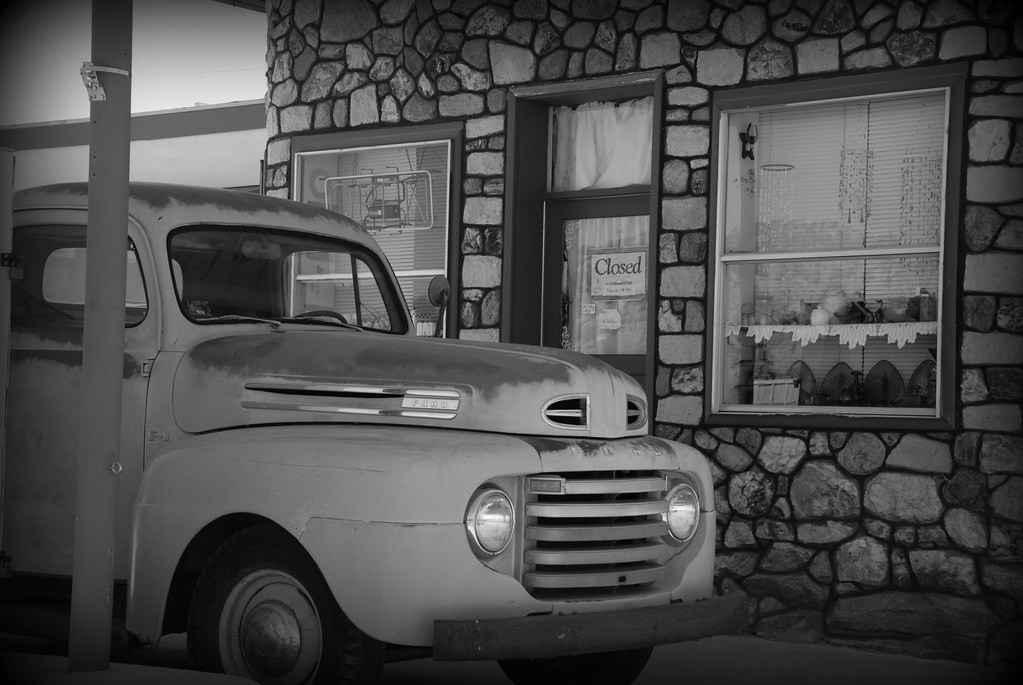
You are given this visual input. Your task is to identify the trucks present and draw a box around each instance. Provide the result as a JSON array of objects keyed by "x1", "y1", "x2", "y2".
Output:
[{"x1": 3, "y1": 179, "x2": 750, "y2": 685}]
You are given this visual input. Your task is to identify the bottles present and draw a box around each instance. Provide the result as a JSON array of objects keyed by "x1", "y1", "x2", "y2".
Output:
[{"x1": 416, "y1": 320, "x2": 437, "y2": 337}]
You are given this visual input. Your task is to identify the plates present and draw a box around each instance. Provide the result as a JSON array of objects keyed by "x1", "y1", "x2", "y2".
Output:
[{"x1": 785, "y1": 359, "x2": 937, "y2": 407}]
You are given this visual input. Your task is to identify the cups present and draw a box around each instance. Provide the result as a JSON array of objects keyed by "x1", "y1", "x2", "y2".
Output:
[
  {"x1": 741, "y1": 310, "x2": 797, "y2": 327},
  {"x1": 810, "y1": 309, "x2": 829, "y2": 325}
]
